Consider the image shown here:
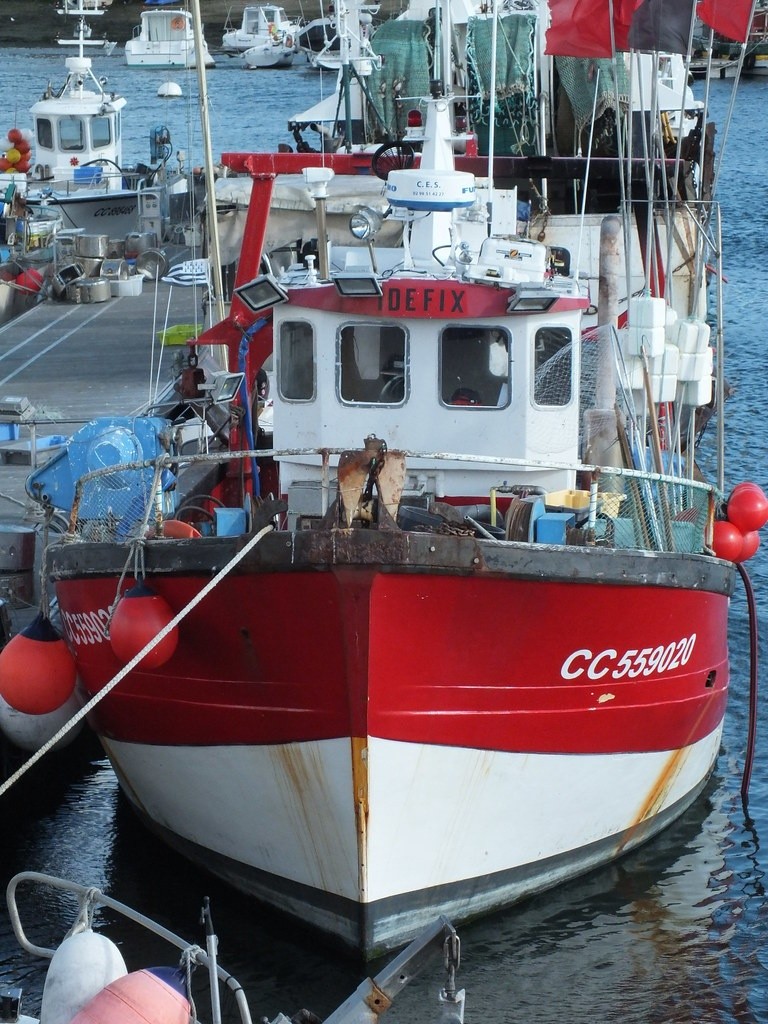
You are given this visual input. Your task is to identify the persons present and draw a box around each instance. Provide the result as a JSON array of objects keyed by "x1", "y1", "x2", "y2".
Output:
[
  {"x1": 302, "y1": 238, "x2": 317, "y2": 267},
  {"x1": 179, "y1": 352, "x2": 210, "y2": 399}
]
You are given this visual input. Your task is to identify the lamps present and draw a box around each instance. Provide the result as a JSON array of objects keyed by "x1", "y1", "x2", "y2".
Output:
[
  {"x1": 506, "y1": 282, "x2": 560, "y2": 314},
  {"x1": 349, "y1": 209, "x2": 381, "y2": 273},
  {"x1": 103, "y1": 40, "x2": 118, "y2": 56},
  {"x1": 73, "y1": 25, "x2": 92, "y2": 38},
  {"x1": 332, "y1": 266, "x2": 384, "y2": 298},
  {"x1": 102, "y1": 91, "x2": 115, "y2": 104},
  {"x1": 233, "y1": 272, "x2": 289, "y2": 314},
  {"x1": 99, "y1": 76, "x2": 108, "y2": 92},
  {"x1": 198, "y1": 370, "x2": 245, "y2": 405}
]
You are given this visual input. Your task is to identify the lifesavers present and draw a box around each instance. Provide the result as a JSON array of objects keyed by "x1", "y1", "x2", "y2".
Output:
[
  {"x1": 171, "y1": 17, "x2": 184, "y2": 30},
  {"x1": 286, "y1": 34, "x2": 293, "y2": 48}
]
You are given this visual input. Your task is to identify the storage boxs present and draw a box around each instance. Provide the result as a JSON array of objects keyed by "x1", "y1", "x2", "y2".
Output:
[
  {"x1": 514, "y1": 489, "x2": 627, "y2": 521},
  {"x1": 52, "y1": 167, "x2": 102, "y2": 184},
  {"x1": 109, "y1": 274, "x2": 145, "y2": 296}
]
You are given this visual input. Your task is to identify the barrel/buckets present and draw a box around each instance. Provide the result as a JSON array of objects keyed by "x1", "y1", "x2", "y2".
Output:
[{"x1": 398, "y1": 507, "x2": 506, "y2": 540}]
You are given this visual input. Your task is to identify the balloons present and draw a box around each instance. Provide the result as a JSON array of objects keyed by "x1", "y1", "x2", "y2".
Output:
[{"x1": 0, "y1": 127, "x2": 32, "y2": 173}]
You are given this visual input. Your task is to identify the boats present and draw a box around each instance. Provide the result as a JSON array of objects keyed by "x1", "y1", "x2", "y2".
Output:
[{"x1": 1, "y1": 2, "x2": 766, "y2": 955}]
